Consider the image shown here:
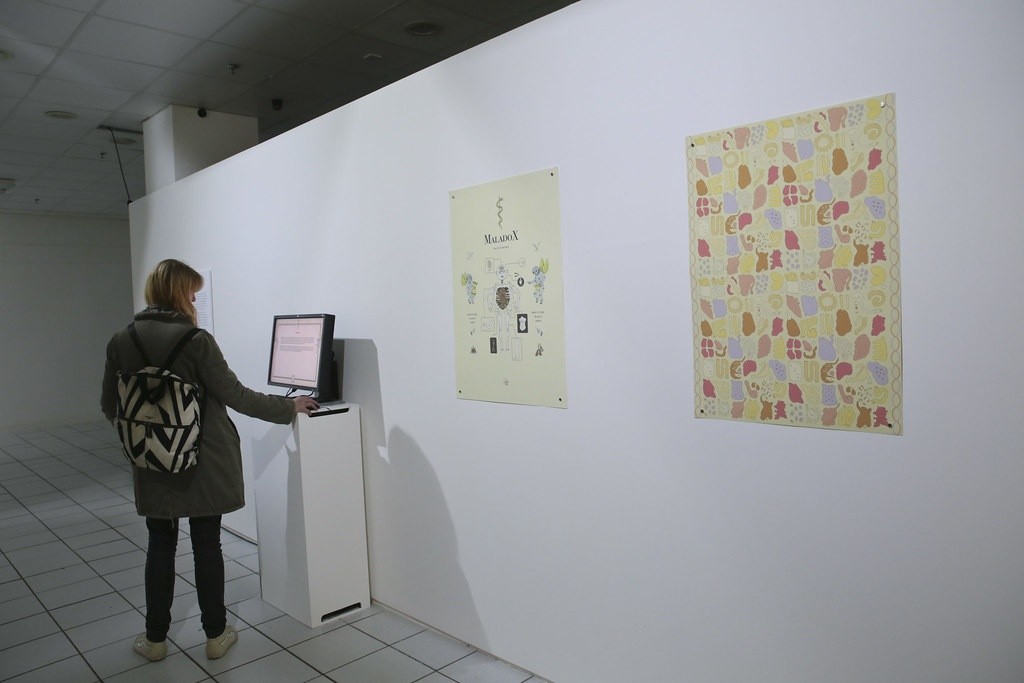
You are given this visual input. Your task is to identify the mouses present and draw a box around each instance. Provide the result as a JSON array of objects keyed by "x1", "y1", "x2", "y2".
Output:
[{"x1": 306, "y1": 404, "x2": 320, "y2": 410}]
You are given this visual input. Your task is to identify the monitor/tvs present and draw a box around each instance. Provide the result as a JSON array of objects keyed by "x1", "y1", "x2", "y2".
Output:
[{"x1": 267, "y1": 314, "x2": 340, "y2": 406}]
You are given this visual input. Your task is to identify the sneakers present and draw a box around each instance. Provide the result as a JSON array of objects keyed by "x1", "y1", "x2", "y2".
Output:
[
  {"x1": 205, "y1": 625, "x2": 238, "y2": 659},
  {"x1": 132, "y1": 632, "x2": 167, "y2": 661}
]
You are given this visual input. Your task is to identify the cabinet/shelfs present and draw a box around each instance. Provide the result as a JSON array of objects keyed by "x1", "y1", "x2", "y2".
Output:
[{"x1": 253, "y1": 402, "x2": 372, "y2": 628}]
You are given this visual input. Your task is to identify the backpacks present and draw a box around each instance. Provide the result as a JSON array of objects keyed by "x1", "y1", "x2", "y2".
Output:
[{"x1": 114, "y1": 322, "x2": 204, "y2": 474}]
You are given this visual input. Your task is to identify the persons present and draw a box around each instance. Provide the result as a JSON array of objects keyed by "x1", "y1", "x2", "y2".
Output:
[{"x1": 100, "y1": 259, "x2": 321, "y2": 663}]
